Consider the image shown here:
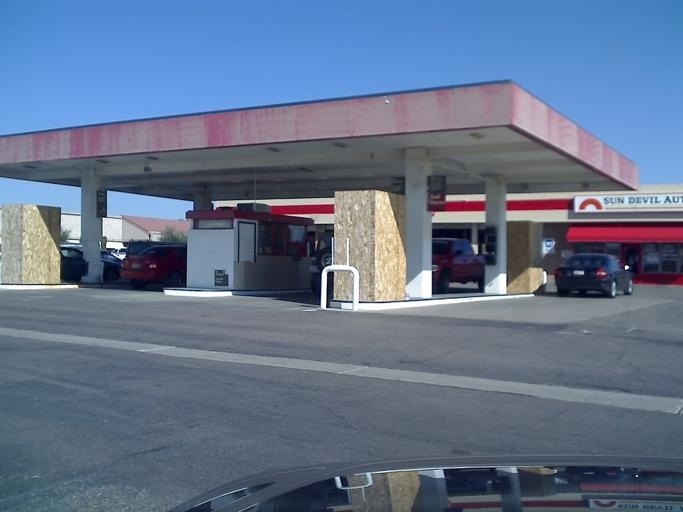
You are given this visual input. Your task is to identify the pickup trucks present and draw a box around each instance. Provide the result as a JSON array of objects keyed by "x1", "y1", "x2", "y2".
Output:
[
  {"x1": 430, "y1": 237, "x2": 484, "y2": 293},
  {"x1": 581, "y1": 242, "x2": 632, "y2": 270}
]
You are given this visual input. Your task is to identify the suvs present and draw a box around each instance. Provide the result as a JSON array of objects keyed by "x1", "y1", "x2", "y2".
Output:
[{"x1": 312, "y1": 231, "x2": 332, "y2": 269}]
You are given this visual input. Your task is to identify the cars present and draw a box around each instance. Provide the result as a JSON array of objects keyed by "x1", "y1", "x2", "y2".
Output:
[
  {"x1": 554, "y1": 254, "x2": 633, "y2": 298},
  {"x1": 170, "y1": 457, "x2": 683, "y2": 511},
  {"x1": 637, "y1": 254, "x2": 682, "y2": 273},
  {"x1": 59, "y1": 244, "x2": 187, "y2": 288}
]
